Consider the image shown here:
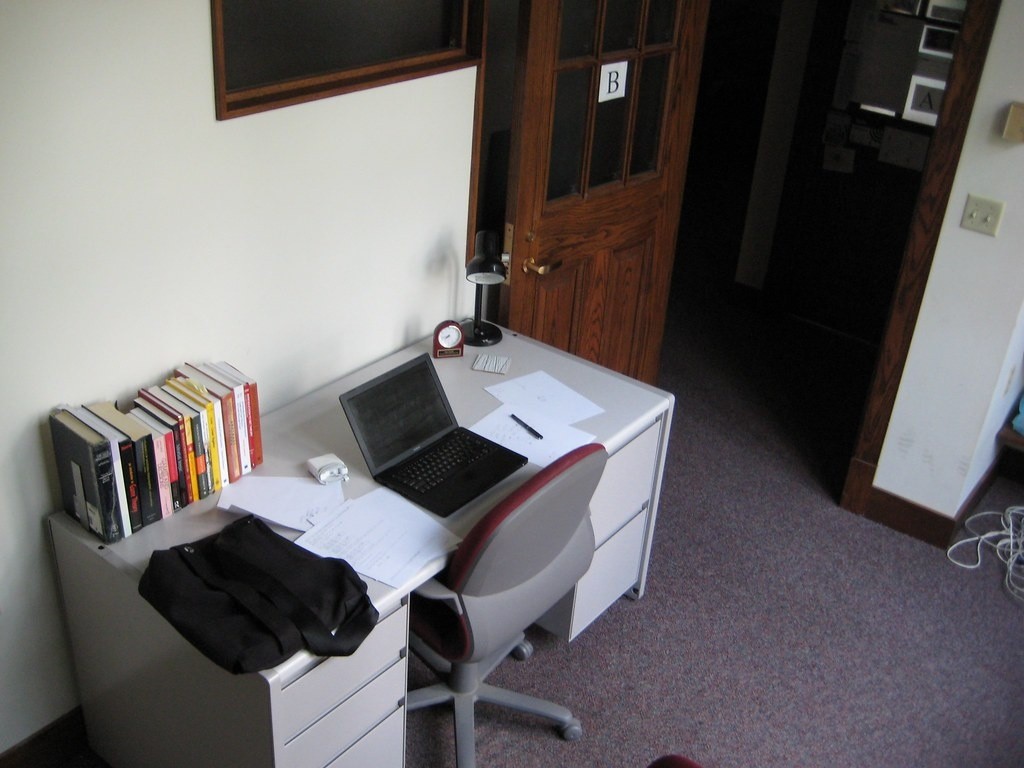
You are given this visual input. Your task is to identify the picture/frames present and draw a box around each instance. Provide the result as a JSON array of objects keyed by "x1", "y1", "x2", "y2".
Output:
[{"x1": 210, "y1": 0, "x2": 486, "y2": 122}]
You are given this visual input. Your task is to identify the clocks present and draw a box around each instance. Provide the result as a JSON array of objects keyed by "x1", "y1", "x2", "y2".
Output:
[{"x1": 433, "y1": 321, "x2": 462, "y2": 357}]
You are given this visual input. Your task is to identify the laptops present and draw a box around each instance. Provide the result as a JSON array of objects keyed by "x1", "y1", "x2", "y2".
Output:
[{"x1": 339, "y1": 353, "x2": 529, "y2": 518}]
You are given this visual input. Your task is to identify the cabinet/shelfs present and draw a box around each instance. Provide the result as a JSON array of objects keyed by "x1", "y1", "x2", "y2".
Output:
[{"x1": 47, "y1": 318, "x2": 676, "y2": 768}]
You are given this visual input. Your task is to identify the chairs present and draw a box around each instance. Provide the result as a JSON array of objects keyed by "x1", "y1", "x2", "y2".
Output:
[{"x1": 407, "y1": 442, "x2": 606, "y2": 768}]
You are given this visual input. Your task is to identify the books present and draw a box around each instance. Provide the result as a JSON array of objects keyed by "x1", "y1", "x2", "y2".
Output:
[{"x1": 49, "y1": 359, "x2": 263, "y2": 545}]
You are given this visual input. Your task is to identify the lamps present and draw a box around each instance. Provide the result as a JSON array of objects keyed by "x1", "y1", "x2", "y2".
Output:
[{"x1": 462, "y1": 230, "x2": 507, "y2": 347}]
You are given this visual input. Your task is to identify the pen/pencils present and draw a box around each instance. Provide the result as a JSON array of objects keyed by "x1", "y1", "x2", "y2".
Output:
[{"x1": 508, "y1": 413, "x2": 543, "y2": 441}]
